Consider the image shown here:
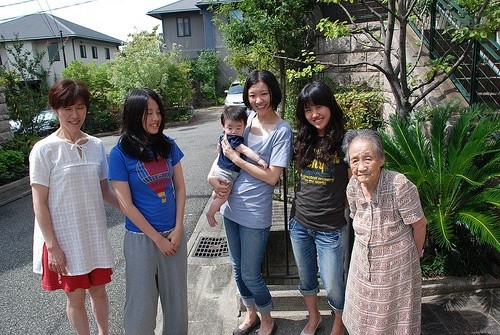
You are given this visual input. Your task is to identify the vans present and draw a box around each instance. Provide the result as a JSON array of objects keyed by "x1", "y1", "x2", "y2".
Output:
[{"x1": 224, "y1": 79, "x2": 249, "y2": 111}]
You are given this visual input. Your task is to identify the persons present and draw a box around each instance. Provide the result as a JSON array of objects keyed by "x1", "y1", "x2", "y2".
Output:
[
  {"x1": 206, "y1": 69, "x2": 294, "y2": 334},
  {"x1": 339, "y1": 129, "x2": 428, "y2": 335},
  {"x1": 28, "y1": 77, "x2": 121, "y2": 335},
  {"x1": 107, "y1": 87, "x2": 188, "y2": 334},
  {"x1": 205, "y1": 103, "x2": 270, "y2": 227},
  {"x1": 288, "y1": 81, "x2": 352, "y2": 335}
]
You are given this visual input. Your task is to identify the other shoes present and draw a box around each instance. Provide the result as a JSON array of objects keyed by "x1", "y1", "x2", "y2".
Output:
[
  {"x1": 233, "y1": 315, "x2": 260, "y2": 335},
  {"x1": 254, "y1": 320, "x2": 278, "y2": 335},
  {"x1": 299, "y1": 315, "x2": 323, "y2": 335}
]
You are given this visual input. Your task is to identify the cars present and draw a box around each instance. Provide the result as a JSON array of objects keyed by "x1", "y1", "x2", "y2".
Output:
[{"x1": 9, "y1": 107, "x2": 60, "y2": 138}]
